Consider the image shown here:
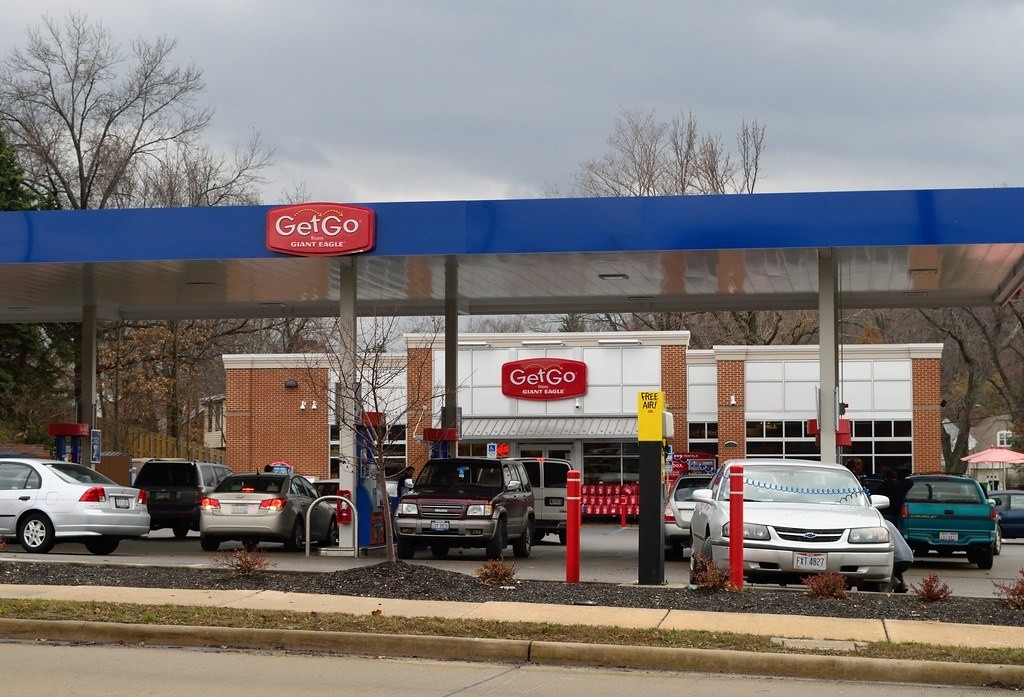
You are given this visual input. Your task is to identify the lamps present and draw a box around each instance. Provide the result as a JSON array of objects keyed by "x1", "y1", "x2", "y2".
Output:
[
  {"x1": 81, "y1": 475, "x2": 93, "y2": 483},
  {"x1": 284, "y1": 381, "x2": 298, "y2": 387},
  {"x1": 311, "y1": 400, "x2": 318, "y2": 409},
  {"x1": 730, "y1": 395, "x2": 736, "y2": 405},
  {"x1": 940, "y1": 399, "x2": 947, "y2": 407},
  {"x1": 299, "y1": 401, "x2": 306, "y2": 409},
  {"x1": 575, "y1": 398, "x2": 580, "y2": 409}
]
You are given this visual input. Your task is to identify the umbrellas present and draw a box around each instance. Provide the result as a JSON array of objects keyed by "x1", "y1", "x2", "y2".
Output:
[{"x1": 959, "y1": 445, "x2": 1024, "y2": 491}]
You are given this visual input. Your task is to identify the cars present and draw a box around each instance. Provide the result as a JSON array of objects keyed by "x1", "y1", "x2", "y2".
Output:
[
  {"x1": 307, "y1": 478, "x2": 339, "y2": 511},
  {"x1": 979, "y1": 489, "x2": 1024, "y2": 539},
  {"x1": 198, "y1": 472, "x2": 338, "y2": 553},
  {"x1": 689, "y1": 459, "x2": 895, "y2": 592},
  {"x1": 0, "y1": 457, "x2": 151, "y2": 555},
  {"x1": 663, "y1": 468, "x2": 715, "y2": 561},
  {"x1": 584, "y1": 446, "x2": 640, "y2": 485}
]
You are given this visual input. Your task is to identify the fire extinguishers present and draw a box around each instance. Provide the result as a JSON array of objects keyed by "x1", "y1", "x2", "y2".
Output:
[{"x1": 339, "y1": 493, "x2": 348, "y2": 520}]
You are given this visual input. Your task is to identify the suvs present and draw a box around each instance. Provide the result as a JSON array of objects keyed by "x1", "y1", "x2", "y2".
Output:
[
  {"x1": 392, "y1": 456, "x2": 537, "y2": 563},
  {"x1": 131, "y1": 459, "x2": 236, "y2": 538},
  {"x1": 505, "y1": 456, "x2": 575, "y2": 545}
]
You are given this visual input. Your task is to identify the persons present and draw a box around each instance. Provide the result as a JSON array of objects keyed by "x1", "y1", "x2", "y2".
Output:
[{"x1": 397, "y1": 467, "x2": 415, "y2": 496}]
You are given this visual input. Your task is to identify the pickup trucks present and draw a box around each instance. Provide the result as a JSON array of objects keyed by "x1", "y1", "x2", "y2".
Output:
[{"x1": 859, "y1": 470, "x2": 1002, "y2": 570}]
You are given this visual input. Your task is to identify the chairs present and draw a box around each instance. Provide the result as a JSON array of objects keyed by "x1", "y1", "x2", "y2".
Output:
[
  {"x1": 267, "y1": 486, "x2": 278, "y2": 491},
  {"x1": 231, "y1": 485, "x2": 241, "y2": 490}
]
